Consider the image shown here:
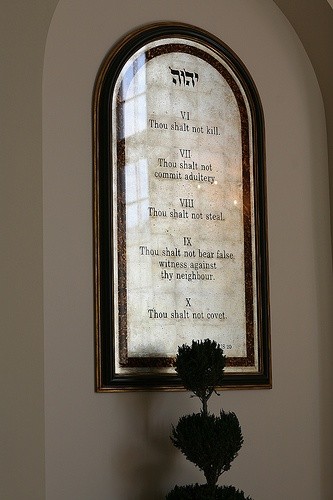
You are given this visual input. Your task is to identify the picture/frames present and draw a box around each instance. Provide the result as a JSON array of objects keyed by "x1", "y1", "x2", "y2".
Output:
[{"x1": 91, "y1": 20, "x2": 273, "y2": 391}]
